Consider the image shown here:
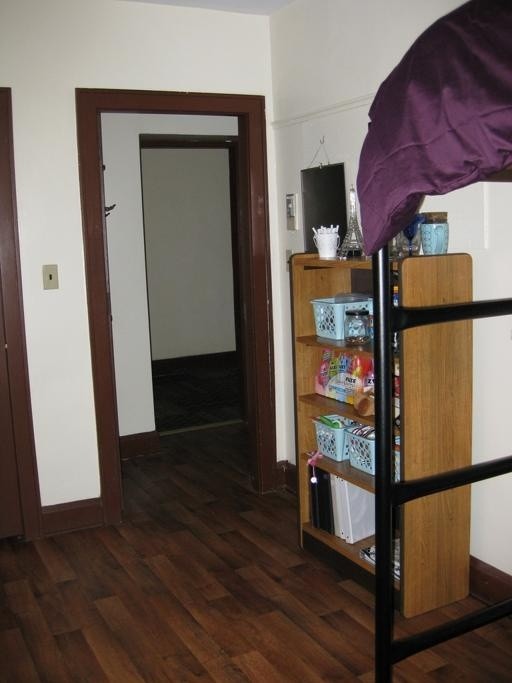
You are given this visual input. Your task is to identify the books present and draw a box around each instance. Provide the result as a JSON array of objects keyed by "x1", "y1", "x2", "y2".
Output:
[{"x1": 360, "y1": 537, "x2": 400, "y2": 580}]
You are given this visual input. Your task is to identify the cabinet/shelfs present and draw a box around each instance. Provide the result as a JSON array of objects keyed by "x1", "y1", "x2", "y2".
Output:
[{"x1": 288, "y1": 251, "x2": 474, "y2": 619}]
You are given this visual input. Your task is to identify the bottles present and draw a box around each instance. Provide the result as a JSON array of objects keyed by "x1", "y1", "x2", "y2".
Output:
[{"x1": 343, "y1": 310, "x2": 370, "y2": 345}]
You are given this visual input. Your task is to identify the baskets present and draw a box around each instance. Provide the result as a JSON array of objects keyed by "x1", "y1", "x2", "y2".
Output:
[
  {"x1": 344, "y1": 428, "x2": 376, "y2": 475},
  {"x1": 314, "y1": 414, "x2": 354, "y2": 461},
  {"x1": 310, "y1": 296, "x2": 373, "y2": 341}
]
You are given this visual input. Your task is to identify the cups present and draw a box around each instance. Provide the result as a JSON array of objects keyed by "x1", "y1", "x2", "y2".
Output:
[
  {"x1": 313, "y1": 234, "x2": 341, "y2": 259},
  {"x1": 420, "y1": 223, "x2": 449, "y2": 256}
]
